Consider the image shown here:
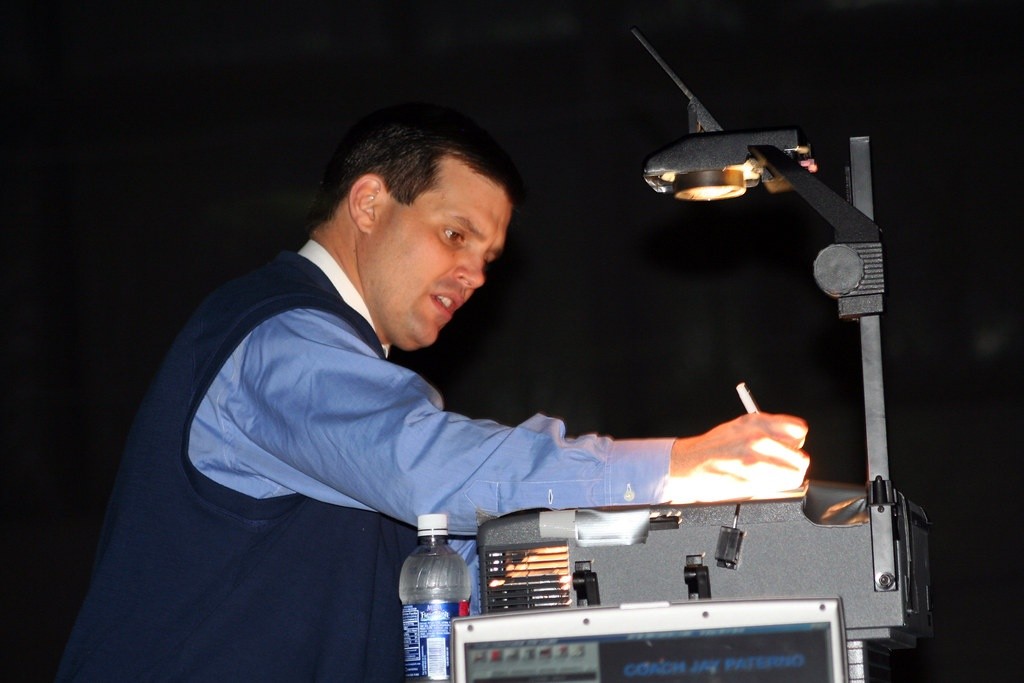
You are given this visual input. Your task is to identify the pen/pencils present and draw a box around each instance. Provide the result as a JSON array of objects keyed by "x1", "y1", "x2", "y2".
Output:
[{"x1": 735, "y1": 382, "x2": 762, "y2": 415}]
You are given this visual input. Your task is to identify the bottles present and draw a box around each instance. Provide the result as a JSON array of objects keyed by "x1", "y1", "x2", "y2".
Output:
[{"x1": 399, "y1": 516, "x2": 472, "y2": 683}]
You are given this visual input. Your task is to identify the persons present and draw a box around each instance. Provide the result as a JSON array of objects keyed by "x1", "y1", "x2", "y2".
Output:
[{"x1": 49, "y1": 105, "x2": 811, "y2": 683}]
